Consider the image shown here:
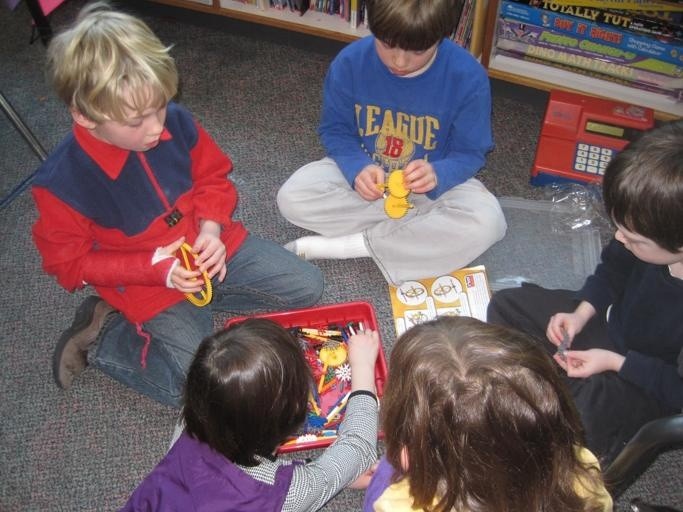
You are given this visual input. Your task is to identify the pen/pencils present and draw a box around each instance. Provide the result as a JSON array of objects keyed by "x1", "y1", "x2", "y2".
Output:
[{"x1": 283, "y1": 325, "x2": 364, "y2": 444}]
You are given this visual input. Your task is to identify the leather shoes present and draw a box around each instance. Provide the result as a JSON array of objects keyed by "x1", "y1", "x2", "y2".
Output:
[{"x1": 54, "y1": 296, "x2": 115, "y2": 389}]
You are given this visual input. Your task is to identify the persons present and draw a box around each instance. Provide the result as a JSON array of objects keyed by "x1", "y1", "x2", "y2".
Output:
[
  {"x1": 276, "y1": 0, "x2": 508, "y2": 286},
  {"x1": 31, "y1": 10, "x2": 325, "y2": 409},
  {"x1": 364, "y1": 316, "x2": 614, "y2": 512},
  {"x1": 119, "y1": 319, "x2": 380, "y2": 512},
  {"x1": 487, "y1": 120, "x2": 683, "y2": 468}
]
